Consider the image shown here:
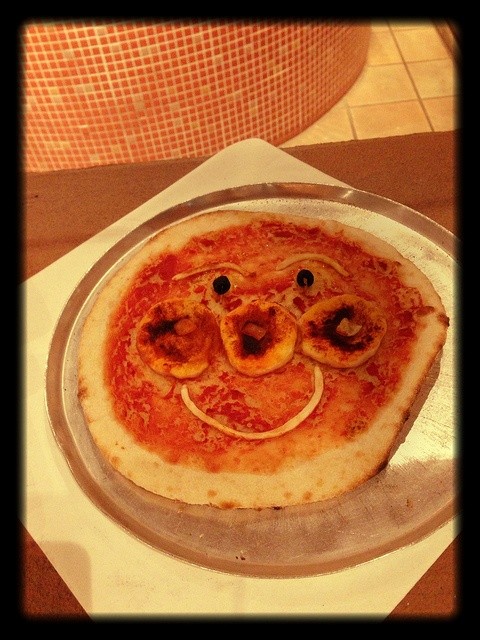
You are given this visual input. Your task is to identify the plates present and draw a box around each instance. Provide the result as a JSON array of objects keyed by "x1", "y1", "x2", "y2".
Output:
[{"x1": 42, "y1": 183, "x2": 458, "y2": 580}]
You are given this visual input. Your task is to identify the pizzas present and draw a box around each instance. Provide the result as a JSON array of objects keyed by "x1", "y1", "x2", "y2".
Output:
[{"x1": 76, "y1": 208, "x2": 450, "y2": 510}]
